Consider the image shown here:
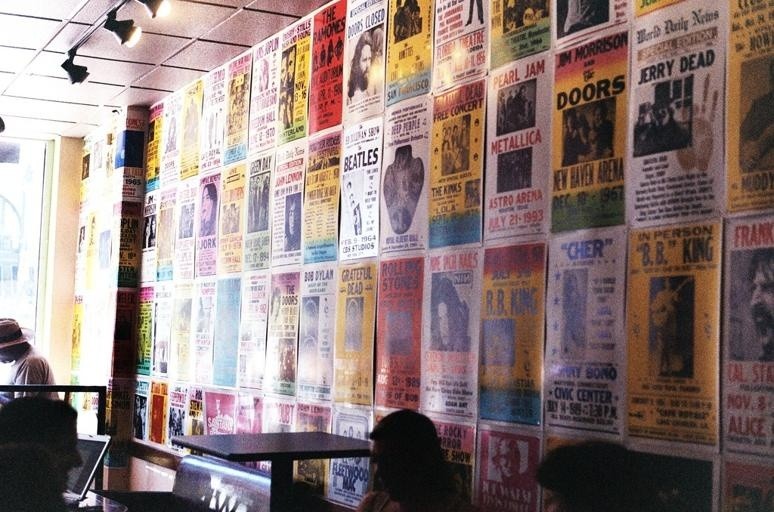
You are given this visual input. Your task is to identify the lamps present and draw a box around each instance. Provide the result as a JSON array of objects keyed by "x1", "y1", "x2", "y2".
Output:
[{"x1": 60, "y1": 0, "x2": 173, "y2": 82}]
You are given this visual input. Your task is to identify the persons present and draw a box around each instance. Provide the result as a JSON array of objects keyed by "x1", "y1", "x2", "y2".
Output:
[
  {"x1": 430, "y1": 275, "x2": 469, "y2": 351},
  {"x1": 285, "y1": 198, "x2": 304, "y2": 250},
  {"x1": 0, "y1": 441, "x2": 69, "y2": 512},
  {"x1": 148, "y1": 216, "x2": 155, "y2": 248},
  {"x1": 198, "y1": 186, "x2": 218, "y2": 236},
  {"x1": 410, "y1": 6, "x2": 422, "y2": 36},
  {"x1": 285, "y1": 92, "x2": 294, "y2": 127},
  {"x1": 439, "y1": 79, "x2": 615, "y2": 208},
  {"x1": 356, "y1": 409, "x2": 464, "y2": 511},
  {"x1": 141, "y1": 218, "x2": 151, "y2": 249},
  {"x1": 492, "y1": 438, "x2": 524, "y2": 491},
  {"x1": 346, "y1": 33, "x2": 378, "y2": 107},
  {"x1": 745, "y1": 248, "x2": 774, "y2": 361},
  {"x1": 393, "y1": 1, "x2": 418, "y2": 42},
  {"x1": 164, "y1": 116, "x2": 176, "y2": 153},
  {"x1": 251, "y1": 177, "x2": 262, "y2": 229},
  {"x1": 269, "y1": 288, "x2": 281, "y2": 320},
  {"x1": 82, "y1": 158, "x2": 89, "y2": 179},
  {"x1": 287, "y1": 46, "x2": 294, "y2": 84},
  {"x1": 465, "y1": 0, "x2": 484, "y2": 27},
  {"x1": 78, "y1": 227, "x2": 85, "y2": 254},
  {"x1": 0, "y1": 396, "x2": 83, "y2": 511},
  {"x1": 281, "y1": 54, "x2": 289, "y2": 90},
  {"x1": 257, "y1": 59, "x2": 268, "y2": 90},
  {"x1": 650, "y1": 276, "x2": 684, "y2": 373},
  {"x1": 0, "y1": 317, "x2": 60, "y2": 402},
  {"x1": 532, "y1": 438, "x2": 667, "y2": 512}
]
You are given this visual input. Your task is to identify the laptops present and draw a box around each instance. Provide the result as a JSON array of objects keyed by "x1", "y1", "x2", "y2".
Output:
[{"x1": 62, "y1": 433, "x2": 111, "y2": 500}]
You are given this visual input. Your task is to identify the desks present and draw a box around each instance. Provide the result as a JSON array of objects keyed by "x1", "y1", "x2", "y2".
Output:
[{"x1": 173, "y1": 432, "x2": 377, "y2": 512}]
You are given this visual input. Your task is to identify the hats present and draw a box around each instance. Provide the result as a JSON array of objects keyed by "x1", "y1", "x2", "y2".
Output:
[
  {"x1": 639, "y1": 102, "x2": 652, "y2": 114},
  {"x1": 685, "y1": 75, "x2": 693, "y2": 96},
  {"x1": 0, "y1": 317, "x2": 34, "y2": 349},
  {"x1": 672, "y1": 79, "x2": 682, "y2": 100},
  {"x1": 652, "y1": 81, "x2": 674, "y2": 107}
]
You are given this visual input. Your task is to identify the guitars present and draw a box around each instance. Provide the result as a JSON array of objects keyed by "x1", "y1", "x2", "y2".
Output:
[{"x1": 651, "y1": 278, "x2": 692, "y2": 330}]
